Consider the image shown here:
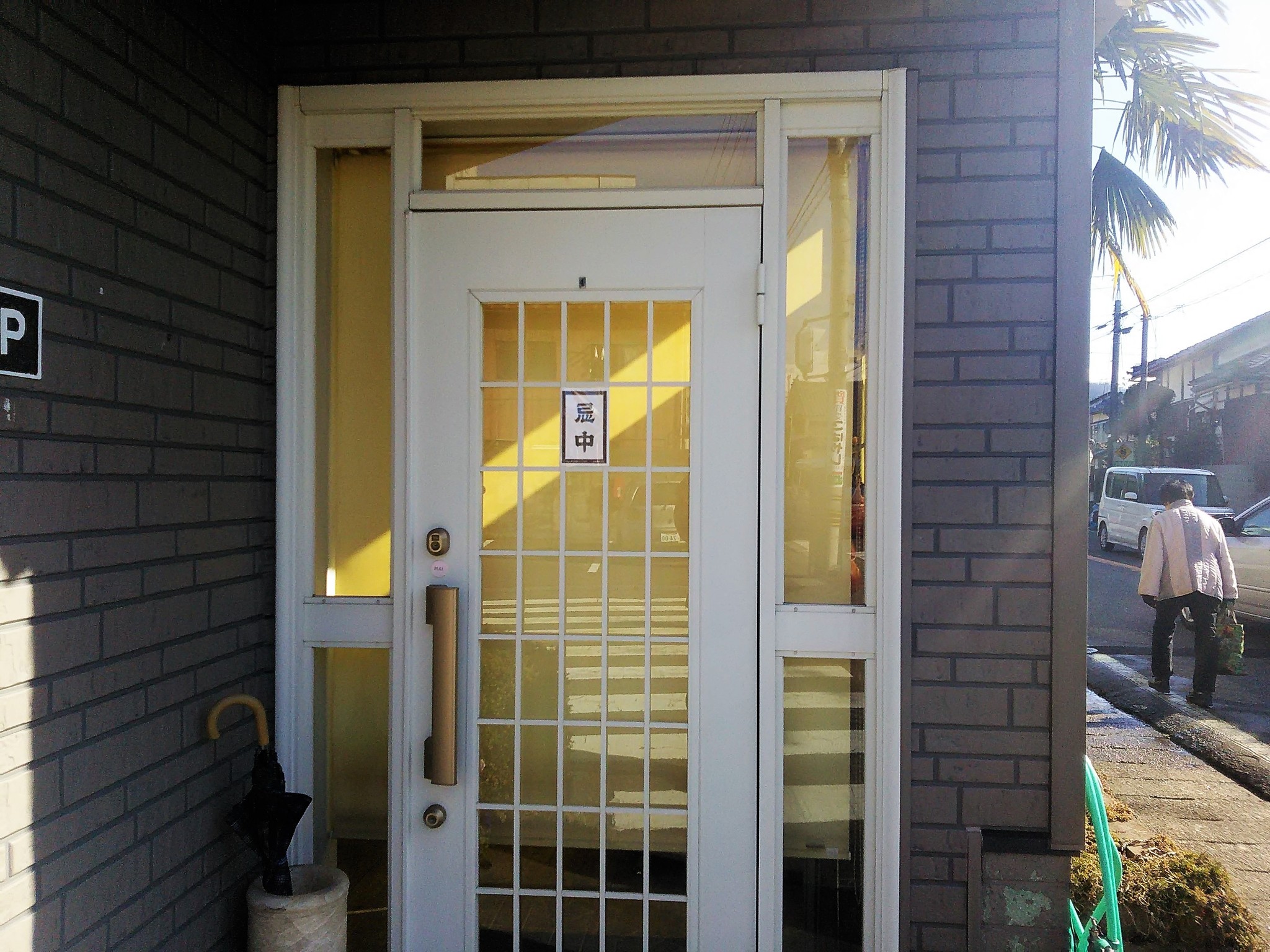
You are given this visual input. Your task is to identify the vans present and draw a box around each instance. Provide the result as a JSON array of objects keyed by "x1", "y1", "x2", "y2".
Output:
[{"x1": 1096, "y1": 466, "x2": 1235, "y2": 561}]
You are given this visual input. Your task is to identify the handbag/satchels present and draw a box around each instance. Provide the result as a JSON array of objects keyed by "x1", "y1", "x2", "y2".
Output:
[{"x1": 1215, "y1": 604, "x2": 1249, "y2": 676}]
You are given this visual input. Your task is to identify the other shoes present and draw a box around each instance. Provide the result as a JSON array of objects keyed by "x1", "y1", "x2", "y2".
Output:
[
  {"x1": 1148, "y1": 676, "x2": 1170, "y2": 693},
  {"x1": 1186, "y1": 690, "x2": 1212, "y2": 706}
]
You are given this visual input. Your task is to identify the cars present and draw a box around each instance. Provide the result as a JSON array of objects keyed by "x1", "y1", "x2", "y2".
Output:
[{"x1": 1178, "y1": 493, "x2": 1270, "y2": 633}]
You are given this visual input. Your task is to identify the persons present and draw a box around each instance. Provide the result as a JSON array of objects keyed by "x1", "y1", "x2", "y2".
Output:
[{"x1": 1137, "y1": 477, "x2": 1239, "y2": 708}]
